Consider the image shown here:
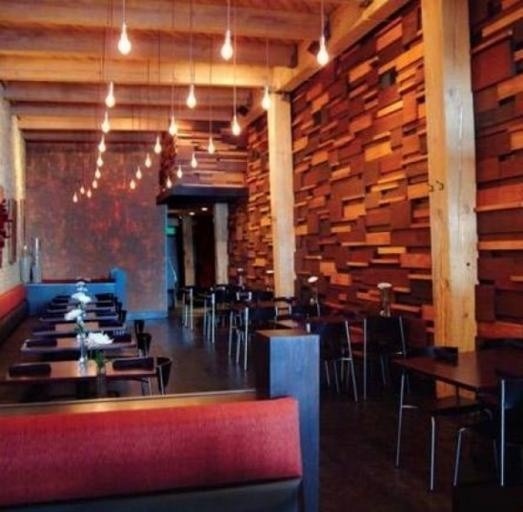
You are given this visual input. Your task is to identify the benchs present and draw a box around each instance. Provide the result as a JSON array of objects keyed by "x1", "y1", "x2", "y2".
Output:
[
  {"x1": 0, "y1": 284, "x2": 42, "y2": 404},
  {"x1": 0, "y1": 329, "x2": 322, "y2": 511}
]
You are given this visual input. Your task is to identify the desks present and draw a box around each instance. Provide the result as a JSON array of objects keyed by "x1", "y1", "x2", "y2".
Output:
[{"x1": 7, "y1": 292, "x2": 172, "y2": 396}]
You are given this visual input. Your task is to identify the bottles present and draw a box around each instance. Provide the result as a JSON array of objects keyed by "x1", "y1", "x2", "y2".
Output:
[{"x1": 20, "y1": 236, "x2": 42, "y2": 284}]
